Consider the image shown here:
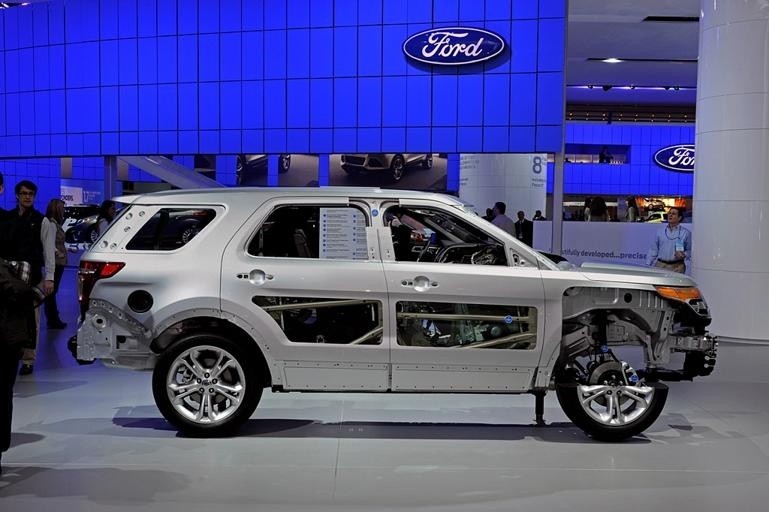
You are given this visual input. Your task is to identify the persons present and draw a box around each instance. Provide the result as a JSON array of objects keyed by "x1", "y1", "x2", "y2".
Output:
[
  {"x1": 481, "y1": 196, "x2": 654, "y2": 248},
  {"x1": 0, "y1": 172, "x2": 117, "y2": 472},
  {"x1": 564, "y1": 158, "x2": 571, "y2": 163},
  {"x1": 599, "y1": 146, "x2": 613, "y2": 163},
  {"x1": 645, "y1": 207, "x2": 691, "y2": 274}
]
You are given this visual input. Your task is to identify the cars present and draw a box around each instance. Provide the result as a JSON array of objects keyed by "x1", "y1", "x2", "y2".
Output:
[
  {"x1": 339, "y1": 152, "x2": 435, "y2": 183},
  {"x1": 70, "y1": 184, "x2": 720, "y2": 442},
  {"x1": 207, "y1": 153, "x2": 291, "y2": 182},
  {"x1": 639, "y1": 196, "x2": 671, "y2": 223},
  {"x1": 59, "y1": 204, "x2": 107, "y2": 253}
]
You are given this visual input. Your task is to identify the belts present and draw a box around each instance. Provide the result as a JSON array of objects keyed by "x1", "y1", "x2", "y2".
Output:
[{"x1": 657, "y1": 259, "x2": 683, "y2": 265}]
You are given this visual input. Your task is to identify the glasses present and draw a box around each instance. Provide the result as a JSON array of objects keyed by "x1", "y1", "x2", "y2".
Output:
[{"x1": 19, "y1": 191, "x2": 36, "y2": 197}]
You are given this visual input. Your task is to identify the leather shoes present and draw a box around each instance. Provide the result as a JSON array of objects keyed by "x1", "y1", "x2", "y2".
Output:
[
  {"x1": 48, "y1": 321, "x2": 67, "y2": 330},
  {"x1": 18, "y1": 363, "x2": 33, "y2": 376}
]
